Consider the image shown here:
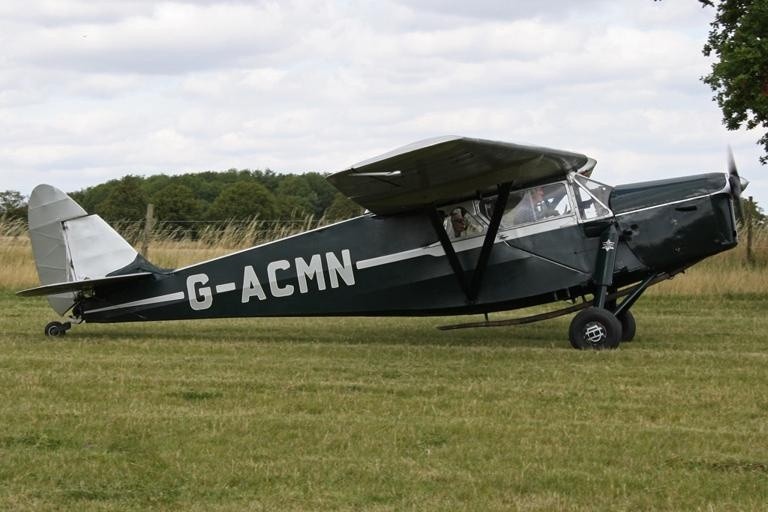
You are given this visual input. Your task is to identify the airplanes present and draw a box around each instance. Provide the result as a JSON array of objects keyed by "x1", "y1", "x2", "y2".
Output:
[{"x1": 15, "y1": 134, "x2": 747, "y2": 353}]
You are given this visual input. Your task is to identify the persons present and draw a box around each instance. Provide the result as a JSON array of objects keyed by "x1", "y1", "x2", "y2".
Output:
[
  {"x1": 513, "y1": 189, "x2": 546, "y2": 225},
  {"x1": 447, "y1": 212, "x2": 465, "y2": 239}
]
